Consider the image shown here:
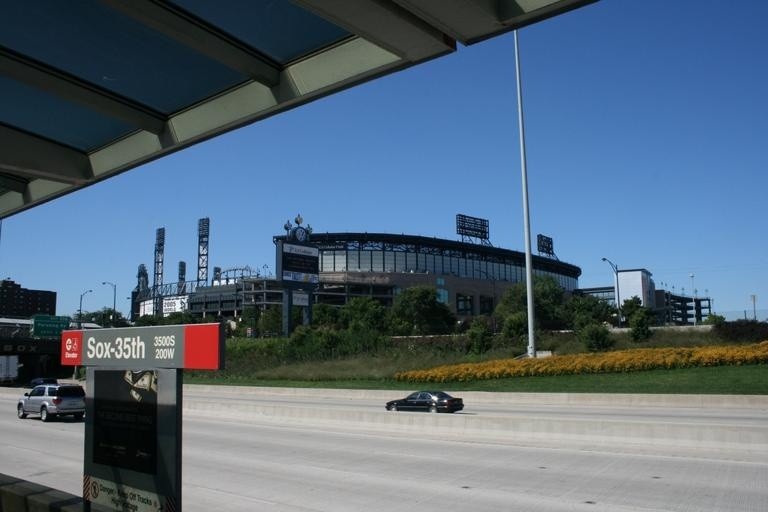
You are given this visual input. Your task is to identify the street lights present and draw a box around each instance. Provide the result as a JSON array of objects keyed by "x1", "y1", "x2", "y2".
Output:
[
  {"x1": 102, "y1": 281, "x2": 117, "y2": 327},
  {"x1": 688, "y1": 273, "x2": 697, "y2": 327},
  {"x1": 474, "y1": 267, "x2": 497, "y2": 337},
  {"x1": 78, "y1": 289, "x2": 93, "y2": 322},
  {"x1": 600, "y1": 257, "x2": 621, "y2": 328}
]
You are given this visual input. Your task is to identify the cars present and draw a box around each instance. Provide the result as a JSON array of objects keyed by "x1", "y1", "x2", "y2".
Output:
[
  {"x1": 23, "y1": 377, "x2": 58, "y2": 388},
  {"x1": 384, "y1": 389, "x2": 464, "y2": 414}
]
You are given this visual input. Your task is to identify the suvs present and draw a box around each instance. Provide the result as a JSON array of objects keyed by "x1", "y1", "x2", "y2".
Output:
[{"x1": 17, "y1": 383, "x2": 86, "y2": 422}]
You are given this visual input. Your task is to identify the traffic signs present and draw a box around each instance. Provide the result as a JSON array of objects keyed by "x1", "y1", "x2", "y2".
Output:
[{"x1": 33, "y1": 314, "x2": 71, "y2": 340}]
